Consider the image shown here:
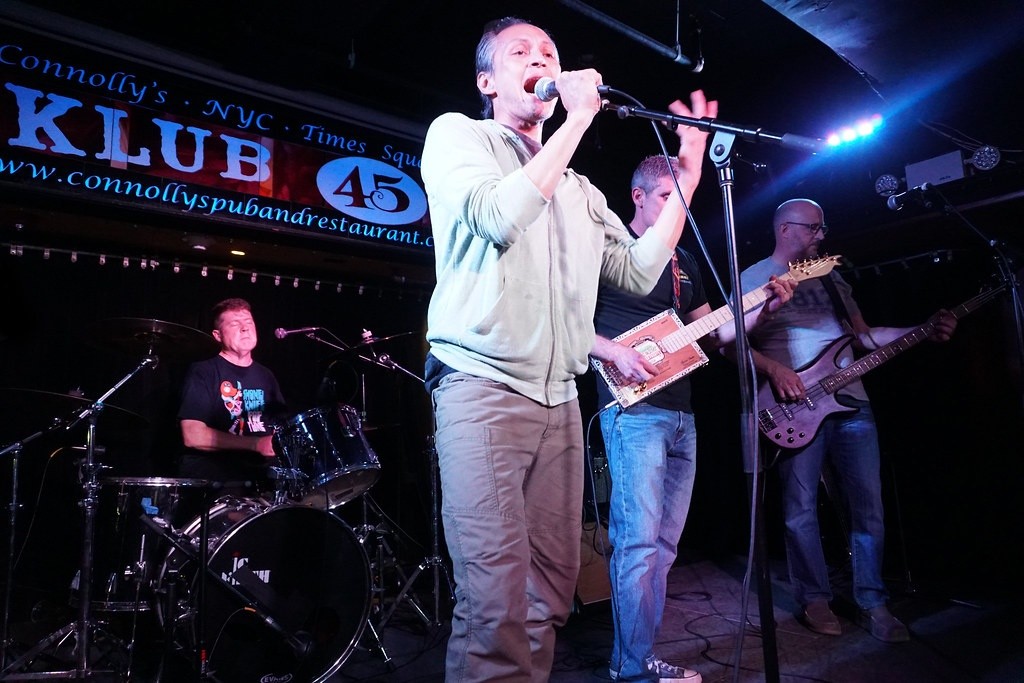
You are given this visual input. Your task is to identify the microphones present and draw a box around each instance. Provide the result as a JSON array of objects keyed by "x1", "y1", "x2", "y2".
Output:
[
  {"x1": 534, "y1": 78, "x2": 611, "y2": 102},
  {"x1": 887, "y1": 182, "x2": 932, "y2": 210},
  {"x1": 275, "y1": 326, "x2": 321, "y2": 339},
  {"x1": 291, "y1": 630, "x2": 315, "y2": 657}
]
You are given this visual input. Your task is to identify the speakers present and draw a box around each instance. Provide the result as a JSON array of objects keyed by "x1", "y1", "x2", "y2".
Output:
[{"x1": 569, "y1": 518, "x2": 616, "y2": 617}]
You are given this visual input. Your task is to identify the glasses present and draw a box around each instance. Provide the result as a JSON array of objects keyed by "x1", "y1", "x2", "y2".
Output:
[{"x1": 786, "y1": 221, "x2": 829, "y2": 235}]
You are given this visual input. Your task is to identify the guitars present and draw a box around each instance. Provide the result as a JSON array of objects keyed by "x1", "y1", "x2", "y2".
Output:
[
  {"x1": 588, "y1": 250, "x2": 843, "y2": 411},
  {"x1": 754, "y1": 280, "x2": 1009, "y2": 451}
]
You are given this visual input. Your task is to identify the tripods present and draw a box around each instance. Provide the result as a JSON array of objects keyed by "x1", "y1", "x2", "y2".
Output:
[
  {"x1": 0, "y1": 335, "x2": 161, "y2": 683},
  {"x1": 353, "y1": 351, "x2": 461, "y2": 652}
]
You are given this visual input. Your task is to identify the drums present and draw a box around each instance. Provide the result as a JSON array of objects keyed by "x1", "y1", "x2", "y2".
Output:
[
  {"x1": 88, "y1": 474, "x2": 228, "y2": 614},
  {"x1": 150, "y1": 492, "x2": 375, "y2": 683},
  {"x1": 271, "y1": 402, "x2": 383, "y2": 511}
]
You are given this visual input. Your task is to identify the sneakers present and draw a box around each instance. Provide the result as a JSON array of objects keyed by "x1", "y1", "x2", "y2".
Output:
[
  {"x1": 796, "y1": 600, "x2": 841, "y2": 634},
  {"x1": 610, "y1": 655, "x2": 702, "y2": 683},
  {"x1": 848, "y1": 607, "x2": 910, "y2": 642}
]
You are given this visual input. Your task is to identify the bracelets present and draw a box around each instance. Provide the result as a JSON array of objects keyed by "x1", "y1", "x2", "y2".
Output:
[{"x1": 251, "y1": 436, "x2": 258, "y2": 452}]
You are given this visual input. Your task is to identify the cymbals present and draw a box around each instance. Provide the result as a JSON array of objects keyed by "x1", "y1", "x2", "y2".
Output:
[
  {"x1": 360, "y1": 425, "x2": 381, "y2": 433},
  {"x1": 21, "y1": 386, "x2": 149, "y2": 424},
  {"x1": 86, "y1": 315, "x2": 224, "y2": 364},
  {"x1": 327, "y1": 331, "x2": 417, "y2": 372}
]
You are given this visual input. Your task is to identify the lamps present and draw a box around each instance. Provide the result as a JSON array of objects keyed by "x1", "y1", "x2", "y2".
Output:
[{"x1": 875, "y1": 145, "x2": 1002, "y2": 197}]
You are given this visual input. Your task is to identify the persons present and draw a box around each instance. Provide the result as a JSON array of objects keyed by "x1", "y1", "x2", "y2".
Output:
[
  {"x1": 723, "y1": 198, "x2": 955, "y2": 645},
  {"x1": 581, "y1": 155, "x2": 799, "y2": 683},
  {"x1": 420, "y1": 15, "x2": 717, "y2": 683},
  {"x1": 168, "y1": 296, "x2": 287, "y2": 497}
]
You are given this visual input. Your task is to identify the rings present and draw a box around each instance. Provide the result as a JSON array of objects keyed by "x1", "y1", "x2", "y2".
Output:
[
  {"x1": 639, "y1": 377, "x2": 643, "y2": 381},
  {"x1": 784, "y1": 290, "x2": 787, "y2": 293}
]
